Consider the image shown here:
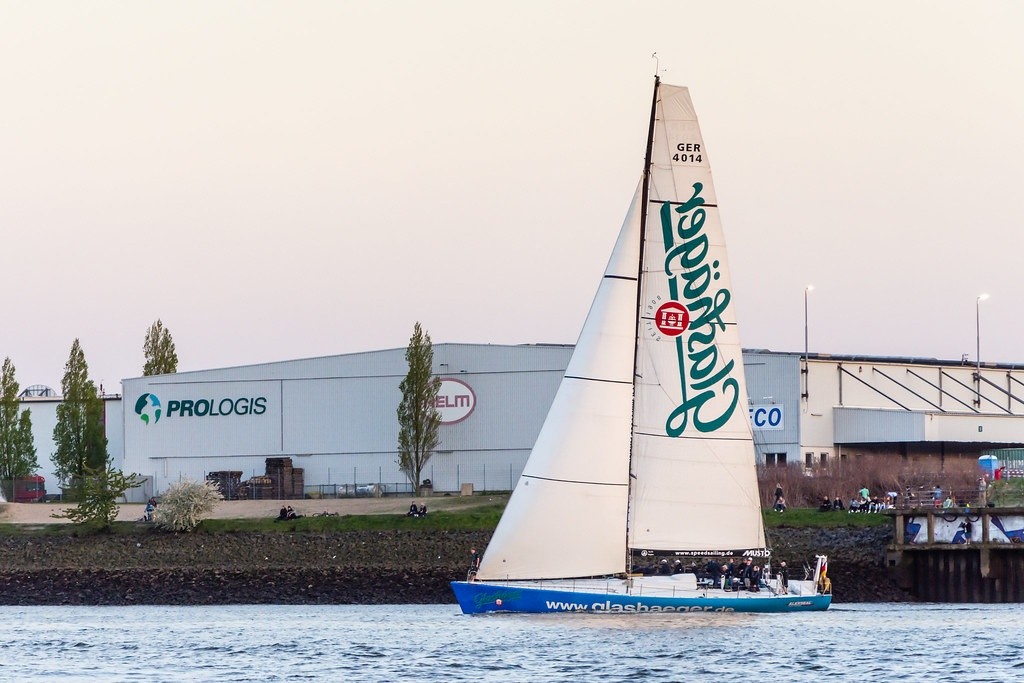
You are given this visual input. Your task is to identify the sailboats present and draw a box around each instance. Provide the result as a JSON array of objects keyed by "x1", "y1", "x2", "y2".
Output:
[{"x1": 450, "y1": 52, "x2": 832, "y2": 615}]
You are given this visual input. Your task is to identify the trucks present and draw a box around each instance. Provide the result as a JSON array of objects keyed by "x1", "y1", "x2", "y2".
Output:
[{"x1": 1, "y1": 476, "x2": 46, "y2": 502}]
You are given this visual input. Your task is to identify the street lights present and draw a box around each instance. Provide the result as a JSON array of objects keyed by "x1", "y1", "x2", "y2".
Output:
[
  {"x1": 801, "y1": 284, "x2": 815, "y2": 401},
  {"x1": 976, "y1": 292, "x2": 991, "y2": 408}
]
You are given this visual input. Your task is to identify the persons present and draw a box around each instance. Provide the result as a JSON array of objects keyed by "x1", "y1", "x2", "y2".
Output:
[
  {"x1": 774, "y1": 496, "x2": 787, "y2": 513},
  {"x1": 817, "y1": 482, "x2": 970, "y2": 514},
  {"x1": 417, "y1": 503, "x2": 427, "y2": 517},
  {"x1": 772, "y1": 483, "x2": 785, "y2": 509},
  {"x1": 144, "y1": 498, "x2": 157, "y2": 523},
  {"x1": 778, "y1": 560, "x2": 789, "y2": 594},
  {"x1": 632, "y1": 556, "x2": 763, "y2": 593},
  {"x1": 273, "y1": 505, "x2": 296, "y2": 523},
  {"x1": 403, "y1": 501, "x2": 418, "y2": 517}
]
